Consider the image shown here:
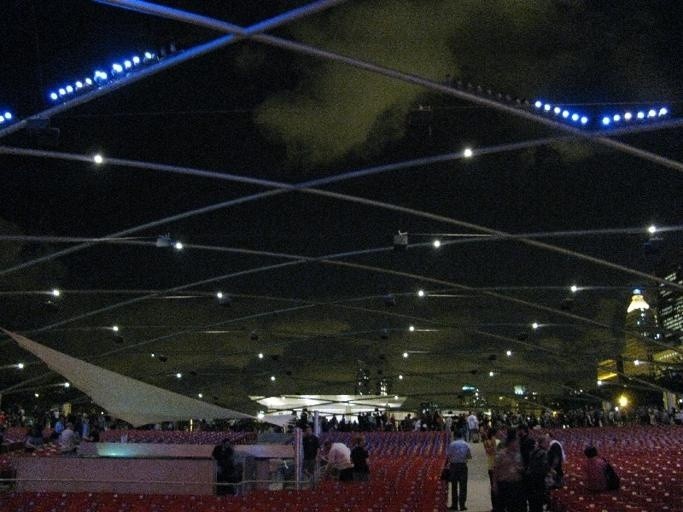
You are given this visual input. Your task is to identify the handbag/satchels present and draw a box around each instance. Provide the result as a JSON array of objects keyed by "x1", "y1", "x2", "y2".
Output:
[
  {"x1": 603, "y1": 456, "x2": 620, "y2": 490},
  {"x1": 441, "y1": 469, "x2": 452, "y2": 482}
]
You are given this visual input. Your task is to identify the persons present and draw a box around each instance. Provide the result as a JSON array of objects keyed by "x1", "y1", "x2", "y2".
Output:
[
  {"x1": 444, "y1": 428, "x2": 472, "y2": 512},
  {"x1": 583, "y1": 446, "x2": 607, "y2": 495},
  {"x1": 484, "y1": 425, "x2": 566, "y2": 511},
  {"x1": 442, "y1": 408, "x2": 683, "y2": 444},
  {"x1": 1, "y1": 407, "x2": 109, "y2": 455},
  {"x1": 213, "y1": 438, "x2": 234, "y2": 494},
  {"x1": 154, "y1": 406, "x2": 444, "y2": 432},
  {"x1": 302, "y1": 426, "x2": 372, "y2": 481}
]
